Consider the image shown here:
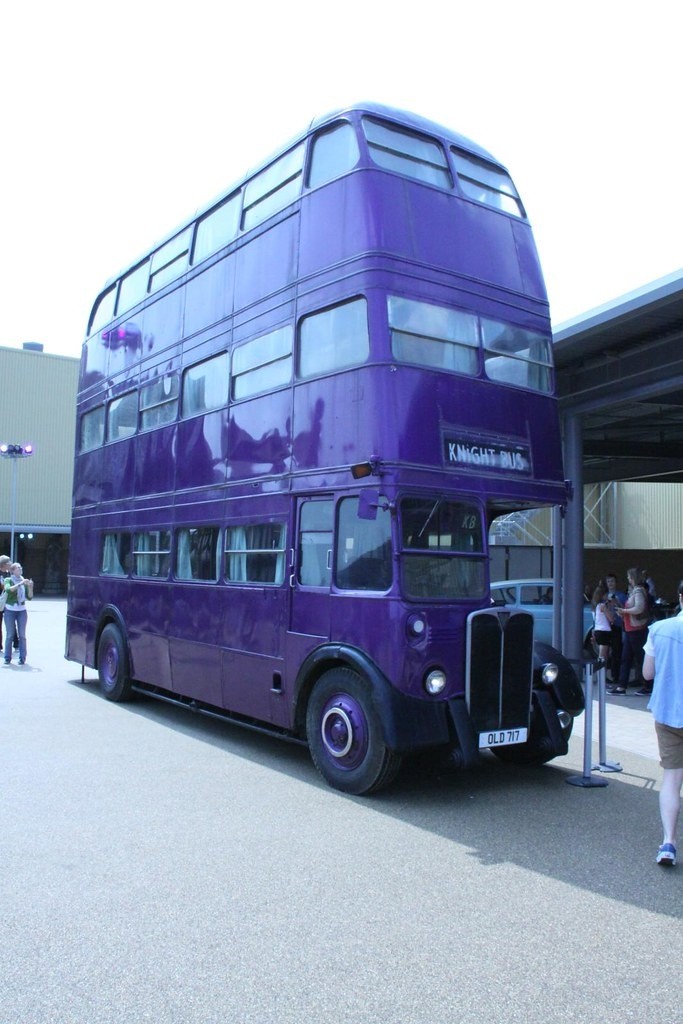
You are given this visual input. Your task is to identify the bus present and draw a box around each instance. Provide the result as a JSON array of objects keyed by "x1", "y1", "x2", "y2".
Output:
[{"x1": 63, "y1": 100, "x2": 575, "y2": 796}]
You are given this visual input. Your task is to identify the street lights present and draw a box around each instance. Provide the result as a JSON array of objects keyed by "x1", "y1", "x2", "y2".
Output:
[{"x1": 0, "y1": 444, "x2": 35, "y2": 564}]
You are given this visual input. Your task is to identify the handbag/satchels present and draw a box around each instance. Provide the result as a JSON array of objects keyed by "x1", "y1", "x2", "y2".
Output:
[
  {"x1": 0, "y1": 589, "x2": 8, "y2": 612},
  {"x1": 633, "y1": 589, "x2": 654, "y2": 620}
]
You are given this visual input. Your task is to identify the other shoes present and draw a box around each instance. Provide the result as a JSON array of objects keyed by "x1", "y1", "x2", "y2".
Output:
[
  {"x1": 606, "y1": 687, "x2": 626, "y2": 696},
  {"x1": 610, "y1": 680, "x2": 619, "y2": 683},
  {"x1": 18, "y1": 658, "x2": 25, "y2": 664},
  {"x1": 629, "y1": 679, "x2": 635, "y2": 683},
  {"x1": 4, "y1": 658, "x2": 11, "y2": 664},
  {"x1": 0, "y1": 644, "x2": 3, "y2": 650},
  {"x1": 14, "y1": 641, "x2": 19, "y2": 648},
  {"x1": 656, "y1": 843, "x2": 676, "y2": 865},
  {"x1": 629, "y1": 682, "x2": 642, "y2": 687},
  {"x1": 635, "y1": 688, "x2": 652, "y2": 696},
  {"x1": 606, "y1": 678, "x2": 613, "y2": 683}
]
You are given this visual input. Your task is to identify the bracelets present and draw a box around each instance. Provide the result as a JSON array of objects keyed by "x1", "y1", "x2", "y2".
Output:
[
  {"x1": 623, "y1": 608, "x2": 627, "y2": 614},
  {"x1": 18, "y1": 582, "x2": 23, "y2": 586}
]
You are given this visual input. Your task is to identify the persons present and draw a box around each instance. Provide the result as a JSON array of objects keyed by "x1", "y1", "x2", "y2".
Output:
[
  {"x1": 536, "y1": 586, "x2": 554, "y2": 605},
  {"x1": 641, "y1": 581, "x2": 683, "y2": 866},
  {"x1": 0, "y1": 555, "x2": 20, "y2": 653},
  {"x1": 3, "y1": 562, "x2": 34, "y2": 664},
  {"x1": 582, "y1": 565, "x2": 659, "y2": 696}
]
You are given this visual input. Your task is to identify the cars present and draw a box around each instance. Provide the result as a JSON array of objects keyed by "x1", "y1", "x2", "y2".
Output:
[{"x1": 488, "y1": 579, "x2": 600, "y2": 660}]
